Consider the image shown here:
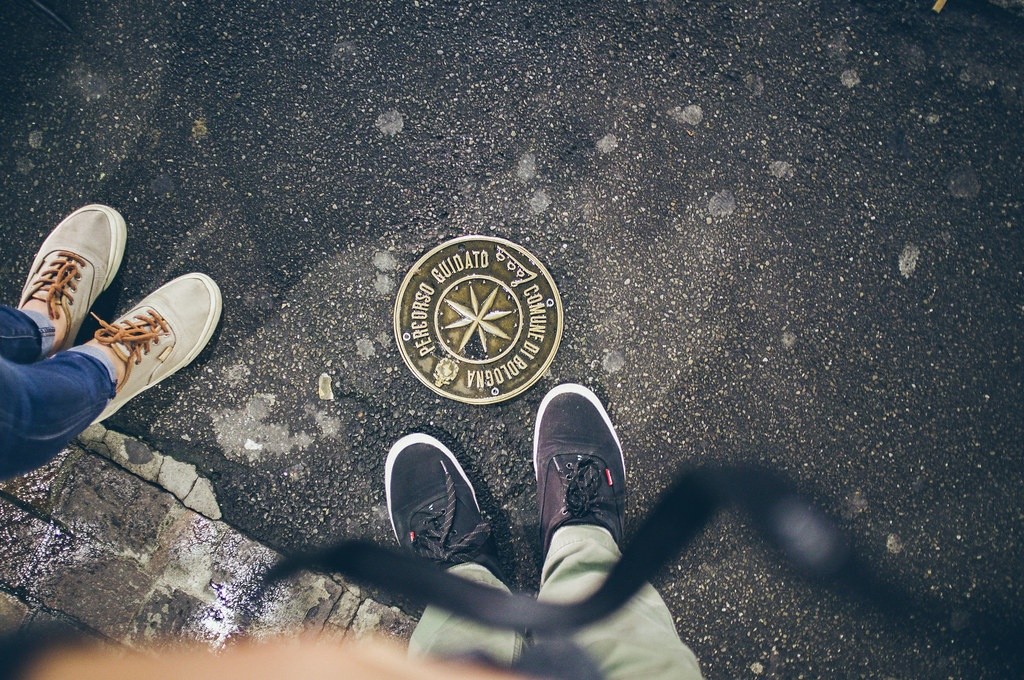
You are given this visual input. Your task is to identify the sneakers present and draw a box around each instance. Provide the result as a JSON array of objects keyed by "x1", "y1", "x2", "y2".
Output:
[
  {"x1": 384, "y1": 434, "x2": 510, "y2": 593},
  {"x1": 49, "y1": 272, "x2": 222, "y2": 425},
  {"x1": 533, "y1": 383, "x2": 627, "y2": 560},
  {"x1": 17, "y1": 204, "x2": 127, "y2": 352}
]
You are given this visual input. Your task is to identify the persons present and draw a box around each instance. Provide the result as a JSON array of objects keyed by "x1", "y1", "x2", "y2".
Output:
[
  {"x1": 0, "y1": 382, "x2": 713, "y2": 680},
  {"x1": 0, "y1": 201, "x2": 224, "y2": 483}
]
